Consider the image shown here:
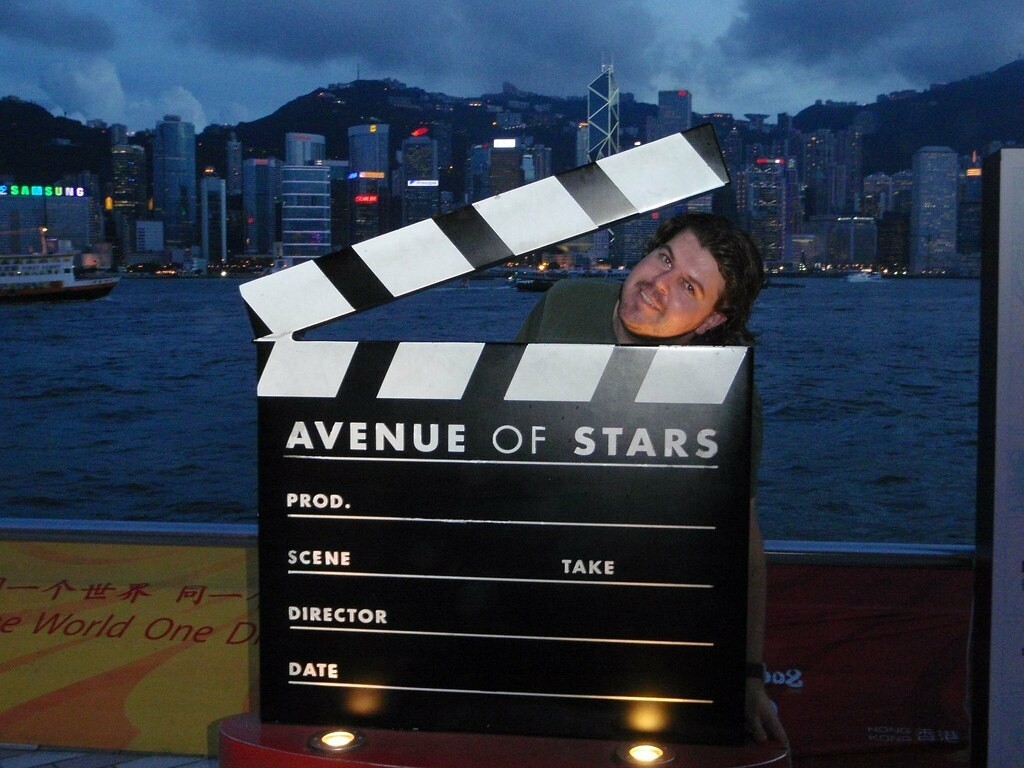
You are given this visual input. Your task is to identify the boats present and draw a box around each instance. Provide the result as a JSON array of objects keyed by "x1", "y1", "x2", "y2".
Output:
[
  {"x1": 504, "y1": 270, "x2": 553, "y2": 293},
  {"x1": 0, "y1": 250, "x2": 119, "y2": 306}
]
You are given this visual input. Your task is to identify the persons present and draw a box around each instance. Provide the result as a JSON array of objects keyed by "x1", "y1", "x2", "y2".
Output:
[{"x1": 512, "y1": 208, "x2": 794, "y2": 768}]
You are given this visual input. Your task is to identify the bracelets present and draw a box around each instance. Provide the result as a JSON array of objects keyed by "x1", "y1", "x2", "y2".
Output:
[{"x1": 742, "y1": 660, "x2": 767, "y2": 680}]
